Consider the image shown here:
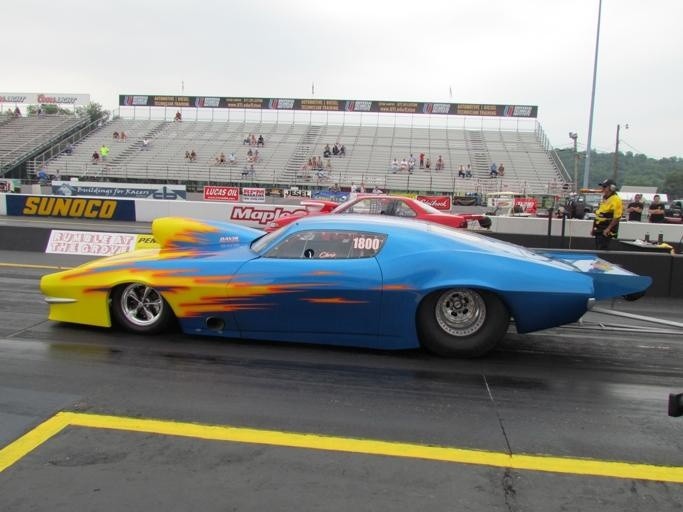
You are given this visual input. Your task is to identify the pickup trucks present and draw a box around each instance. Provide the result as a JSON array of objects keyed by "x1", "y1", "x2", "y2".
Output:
[{"x1": 565, "y1": 193, "x2": 602, "y2": 215}]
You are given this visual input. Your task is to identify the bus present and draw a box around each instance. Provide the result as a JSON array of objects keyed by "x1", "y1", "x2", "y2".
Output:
[{"x1": 487, "y1": 191, "x2": 538, "y2": 216}]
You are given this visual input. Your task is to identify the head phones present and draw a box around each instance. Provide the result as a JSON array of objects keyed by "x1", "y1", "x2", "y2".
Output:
[{"x1": 609, "y1": 184, "x2": 621, "y2": 193}]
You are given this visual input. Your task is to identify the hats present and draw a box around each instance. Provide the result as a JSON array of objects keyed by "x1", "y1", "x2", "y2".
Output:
[{"x1": 599, "y1": 179, "x2": 616, "y2": 188}]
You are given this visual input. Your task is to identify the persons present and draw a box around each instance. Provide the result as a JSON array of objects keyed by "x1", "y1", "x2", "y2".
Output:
[
  {"x1": 626, "y1": 193, "x2": 644, "y2": 221},
  {"x1": 0, "y1": 105, "x2": 601, "y2": 220},
  {"x1": 590, "y1": 177, "x2": 624, "y2": 249},
  {"x1": 647, "y1": 194, "x2": 666, "y2": 223}
]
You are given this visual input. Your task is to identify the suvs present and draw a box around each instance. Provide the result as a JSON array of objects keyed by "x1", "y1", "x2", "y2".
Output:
[{"x1": 668, "y1": 199, "x2": 683, "y2": 217}]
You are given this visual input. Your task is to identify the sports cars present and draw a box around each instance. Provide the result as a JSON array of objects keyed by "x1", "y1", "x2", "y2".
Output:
[
  {"x1": 264, "y1": 193, "x2": 491, "y2": 233},
  {"x1": 40, "y1": 216, "x2": 654, "y2": 358}
]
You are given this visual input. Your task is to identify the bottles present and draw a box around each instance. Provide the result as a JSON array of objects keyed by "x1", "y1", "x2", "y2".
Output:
[{"x1": 645, "y1": 229, "x2": 663, "y2": 245}]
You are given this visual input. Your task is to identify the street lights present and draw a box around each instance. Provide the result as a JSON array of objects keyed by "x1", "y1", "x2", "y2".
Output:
[{"x1": 569, "y1": 131, "x2": 578, "y2": 196}]
